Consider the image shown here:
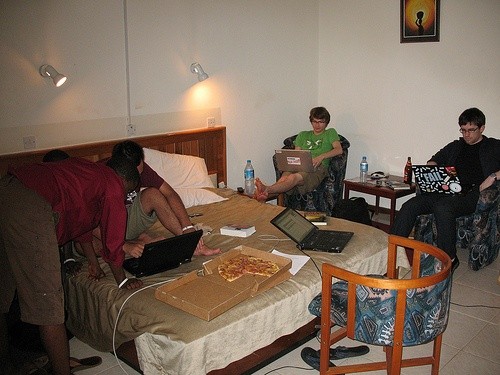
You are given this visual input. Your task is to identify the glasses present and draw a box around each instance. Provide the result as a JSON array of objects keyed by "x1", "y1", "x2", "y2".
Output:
[
  {"x1": 312, "y1": 120, "x2": 326, "y2": 124},
  {"x1": 459, "y1": 127, "x2": 479, "y2": 133}
]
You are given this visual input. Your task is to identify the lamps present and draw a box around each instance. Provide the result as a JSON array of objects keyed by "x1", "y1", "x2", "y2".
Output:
[
  {"x1": 41, "y1": 64, "x2": 66, "y2": 88},
  {"x1": 191, "y1": 63, "x2": 208, "y2": 82}
]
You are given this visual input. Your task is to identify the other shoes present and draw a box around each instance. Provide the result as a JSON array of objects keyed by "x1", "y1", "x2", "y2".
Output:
[
  {"x1": 18, "y1": 355, "x2": 49, "y2": 375},
  {"x1": 451, "y1": 254, "x2": 460, "y2": 273}
]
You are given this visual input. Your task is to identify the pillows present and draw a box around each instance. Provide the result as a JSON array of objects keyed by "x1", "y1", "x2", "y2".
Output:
[
  {"x1": 170, "y1": 186, "x2": 231, "y2": 207},
  {"x1": 141, "y1": 147, "x2": 216, "y2": 189}
]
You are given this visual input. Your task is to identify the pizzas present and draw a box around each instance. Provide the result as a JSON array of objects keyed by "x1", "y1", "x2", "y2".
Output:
[{"x1": 218, "y1": 255, "x2": 279, "y2": 282}]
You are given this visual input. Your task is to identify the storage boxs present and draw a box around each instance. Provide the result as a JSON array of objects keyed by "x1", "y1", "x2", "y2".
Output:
[{"x1": 155, "y1": 245, "x2": 292, "y2": 322}]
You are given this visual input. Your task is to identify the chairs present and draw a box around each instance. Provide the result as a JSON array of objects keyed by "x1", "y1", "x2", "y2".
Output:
[
  {"x1": 410, "y1": 182, "x2": 500, "y2": 271},
  {"x1": 271, "y1": 132, "x2": 352, "y2": 212},
  {"x1": 319, "y1": 235, "x2": 467, "y2": 375}
]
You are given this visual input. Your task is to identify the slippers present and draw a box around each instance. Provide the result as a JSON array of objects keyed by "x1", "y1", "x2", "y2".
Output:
[
  {"x1": 316, "y1": 346, "x2": 370, "y2": 360},
  {"x1": 301, "y1": 347, "x2": 346, "y2": 375},
  {"x1": 70, "y1": 356, "x2": 102, "y2": 372}
]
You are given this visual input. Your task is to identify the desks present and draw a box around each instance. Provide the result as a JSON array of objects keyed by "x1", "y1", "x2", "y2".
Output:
[{"x1": 341, "y1": 175, "x2": 418, "y2": 234}]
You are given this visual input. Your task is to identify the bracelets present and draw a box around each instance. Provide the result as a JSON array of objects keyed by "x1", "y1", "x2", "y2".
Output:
[
  {"x1": 182, "y1": 225, "x2": 194, "y2": 231},
  {"x1": 118, "y1": 278, "x2": 128, "y2": 288},
  {"x1": 63, "y1": 258, "x2": 76, "y2": 263}
]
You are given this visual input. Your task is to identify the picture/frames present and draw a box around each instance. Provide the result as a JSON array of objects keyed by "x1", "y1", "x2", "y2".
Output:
[{"x1": 400, "y1": 0, "x2": 441, "y2": 44}]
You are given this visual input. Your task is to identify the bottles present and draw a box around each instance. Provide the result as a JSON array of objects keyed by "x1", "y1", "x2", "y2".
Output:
[
  {"x1": 244, "y1": 160, "x2": 255, "y2": 194},
  {"x1": 360, "y1": 156, "x2": 368, "y2": 183},
  {"x1": 404, "y1": 157, "x2": 412, "y2": 184}
]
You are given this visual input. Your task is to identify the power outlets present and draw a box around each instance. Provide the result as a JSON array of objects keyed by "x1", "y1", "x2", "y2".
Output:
[
  {"x1": 207, "y1": 117, "x2": 216, "y2": 127},
  {"x1": 22, "y1": 136, "x2": 37, "y2": 151}
]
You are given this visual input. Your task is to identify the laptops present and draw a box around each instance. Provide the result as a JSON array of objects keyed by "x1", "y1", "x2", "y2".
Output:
[
  {"x1": 270, "y1": 206, "x2": 355, "y2": 253},
  {"x1": 410, "y1": 164, "x2": 476, "y2": 196},
  {"x1": 275, "y1": 150, "x2": 319, "y2": 172},
  {"x1": 122, "y1": 229, "x2": 203, "y2": 279}
]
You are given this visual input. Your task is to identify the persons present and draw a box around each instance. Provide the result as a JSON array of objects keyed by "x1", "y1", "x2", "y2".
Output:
[
  {"x1": 75, "y1": 141, "x2": 222, "y2": 257},
  {"x1": 252, "y1": 106, "x2": 343, "y2": 201},
  {"x1": 43, "y1": 150, "x2": 82, "y2": 276},
  {"x1": 393, "y1": 107, "x2": 500, "y2": 274},
  {"x1": 0, "y1": 155, "x2": 143, "y2": 375}
]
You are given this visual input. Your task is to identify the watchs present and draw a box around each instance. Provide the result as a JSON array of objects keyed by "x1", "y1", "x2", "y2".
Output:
[{"x1": 490, "y1": 172, "x2": 497, "y2": 181}]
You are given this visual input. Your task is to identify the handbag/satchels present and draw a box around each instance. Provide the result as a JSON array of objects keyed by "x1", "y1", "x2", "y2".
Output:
[{"x1": 334, "y1": 197, "x2": 375, "y2": 224}]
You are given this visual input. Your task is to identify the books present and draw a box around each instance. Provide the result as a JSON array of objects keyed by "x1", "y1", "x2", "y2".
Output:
[
  {"x1": 304, "y1": 211, "x2": 327, "y2": 225},
  {"x1": 220, "y1": 224, "x2": 257, "y2": 237}
]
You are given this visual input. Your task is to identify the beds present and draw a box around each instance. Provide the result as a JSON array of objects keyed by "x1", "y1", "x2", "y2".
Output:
[{"x1": 0, "y1": 126, "x2": 408, "y2": 373}]
